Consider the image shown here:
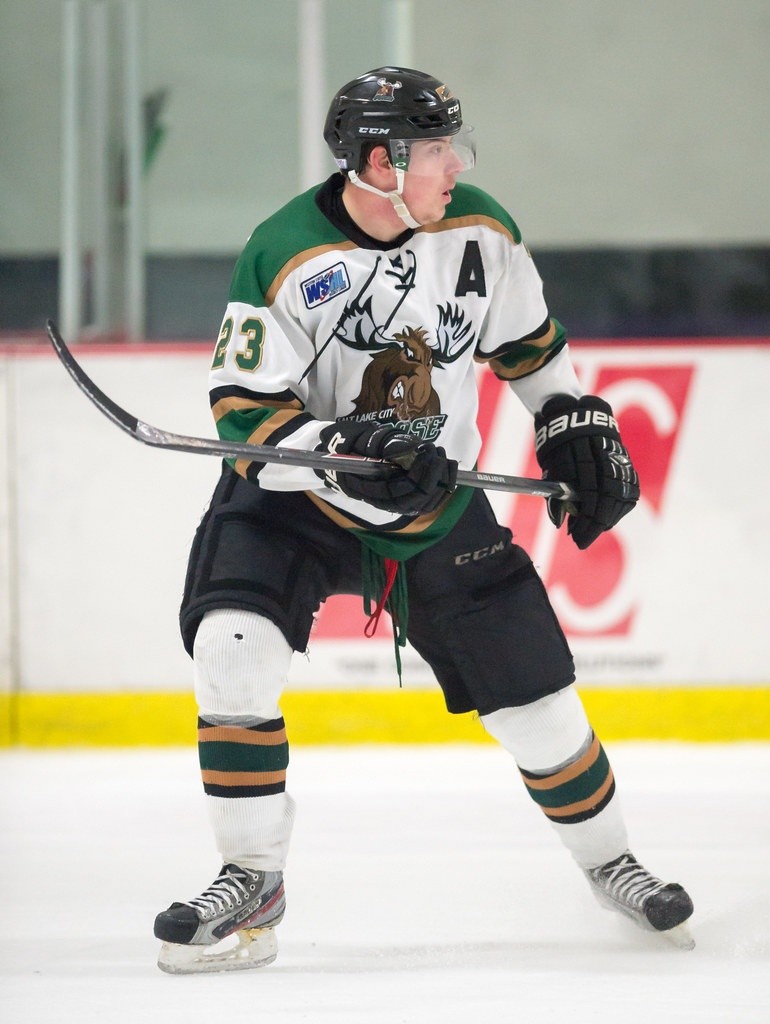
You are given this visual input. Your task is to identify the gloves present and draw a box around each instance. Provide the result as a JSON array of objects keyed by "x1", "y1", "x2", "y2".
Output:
[
  {"x1": 534, "y1": 393, "x2": 641, "y2": 550},
  {"x1": 313, "y1": 420, "x2": 458, "y2": 515}
]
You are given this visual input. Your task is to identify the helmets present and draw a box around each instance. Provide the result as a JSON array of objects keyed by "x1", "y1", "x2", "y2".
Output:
[{"x1": 324, "y1": 65, "x2": 462, "y2": 179}]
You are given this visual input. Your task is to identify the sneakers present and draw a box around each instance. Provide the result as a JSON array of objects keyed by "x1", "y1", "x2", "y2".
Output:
[
  {"x1": 154, "y1": 863, "x2": 286, "y2": 974},
  {"x1": 583, "y1": 849, "x2": 695, "y2": 952}
]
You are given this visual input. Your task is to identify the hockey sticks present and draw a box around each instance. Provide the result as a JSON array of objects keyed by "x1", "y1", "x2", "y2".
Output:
[{"x1": 40, "y1": 318, "x2": 575, "y2": 501}]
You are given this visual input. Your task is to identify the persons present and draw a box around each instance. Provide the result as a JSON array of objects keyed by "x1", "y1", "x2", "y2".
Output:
[{"x1": 154, "y1": 66, "x2": 696, "y2": 973}]
